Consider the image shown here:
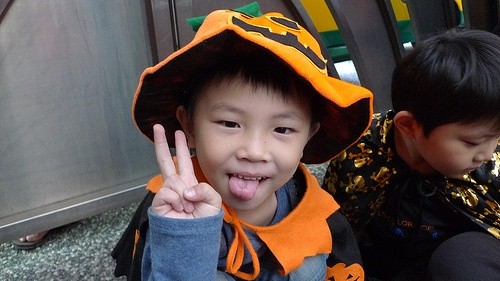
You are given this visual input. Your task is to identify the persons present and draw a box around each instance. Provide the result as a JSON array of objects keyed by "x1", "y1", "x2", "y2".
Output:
[
  {"x1": 321, "y1": 27, "x2": 500, "y2": 281},
  {"x1": 110, "y1": 9, "x2": 374, "y2": 281}
]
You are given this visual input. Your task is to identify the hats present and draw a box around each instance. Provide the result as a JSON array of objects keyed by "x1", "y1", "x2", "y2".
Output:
[{"x1": 132, "y1": 1, "x2": 374, "y2": 165}]
